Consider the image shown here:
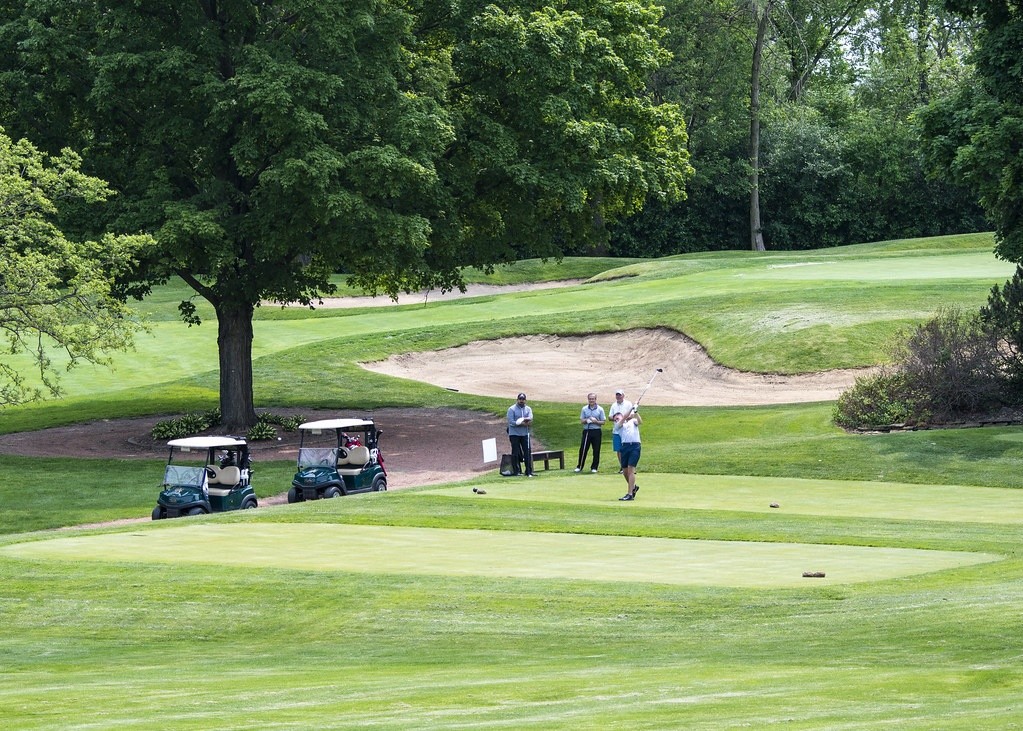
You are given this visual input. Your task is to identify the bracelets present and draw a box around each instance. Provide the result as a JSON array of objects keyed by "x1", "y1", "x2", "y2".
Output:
[{"x1": 634, "y1": 411, "x2": 637, "y2": 413}]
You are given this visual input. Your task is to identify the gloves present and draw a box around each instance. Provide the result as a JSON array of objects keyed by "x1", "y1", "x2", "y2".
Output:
[
  {"x1": 633, "y1": 404, "x2": 638, "y2": 413},
  {"x1": 516, "y1": 418, "x2": 524, "y2": 425},
  {"x1": 587, "y1": 419, "x2": 593, "y2": 424}
]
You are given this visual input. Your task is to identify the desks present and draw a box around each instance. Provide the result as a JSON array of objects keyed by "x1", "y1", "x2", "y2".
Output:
[{"x1": 523, "y1": 450, "x2": 564, "y2": 471}]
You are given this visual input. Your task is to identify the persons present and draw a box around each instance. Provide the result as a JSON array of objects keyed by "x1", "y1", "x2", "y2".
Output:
[
  {"x1": 574, "y1": 393, "x2": 606, "y2": 472},
  {"x1": 613, "y1": 400, "x2": 643, "y2": 501},
  {"x1": 608, "y1": 390, "x2": 633, "y2": 473},
  {"x1": 507, "y1": 393, "x2": 535, "y2": 478}
]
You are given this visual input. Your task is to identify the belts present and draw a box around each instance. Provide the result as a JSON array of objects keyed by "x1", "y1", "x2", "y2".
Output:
[{"x1": 622, "y1": 442, "x2": 640, "y2": 445}]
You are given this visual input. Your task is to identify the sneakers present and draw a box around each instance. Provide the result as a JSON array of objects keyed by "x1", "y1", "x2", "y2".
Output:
[
  {"x1": 619, "y1": 494, "x2": 634, "y2": 501},
  {"x1": 633, "y1": 484, "x2": 639, "y2": 498}
]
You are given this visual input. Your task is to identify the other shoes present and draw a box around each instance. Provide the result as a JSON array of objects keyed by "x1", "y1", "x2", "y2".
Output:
[
  {"x1": 531, "y1": 472, "x2": 538, "y2": 477},
  {"x1": 618, "y1": 470, "x2": 623, "y2": 474},
  {"x1": 574, "y1": 468, "x2": 580, "y2": 473},
  {"x1": 592, "y1": 469, "x2": 596, "y2": 473}
]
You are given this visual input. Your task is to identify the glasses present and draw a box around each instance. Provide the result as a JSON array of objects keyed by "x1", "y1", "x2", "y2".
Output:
[{"x1": 519, "y1": 398, "x2": 525, "y2": 400}]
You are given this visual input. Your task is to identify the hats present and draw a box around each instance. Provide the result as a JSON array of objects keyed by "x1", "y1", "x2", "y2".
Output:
[
  {"x1": 616, "y1": 389, "x2": 624, "y2": 395},
  {"x1": 518, "y1": 393, "x2": 526, "y2": 399}
]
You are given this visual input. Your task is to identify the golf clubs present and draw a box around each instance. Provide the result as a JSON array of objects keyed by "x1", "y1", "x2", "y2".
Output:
[
  {"x1": 368, "y1": 429, "x2": 384, "y2": 448},
  {"x1": 634, "y1": 368, "x2": 663, "y2": 412},
  {"x1": 527, "y1": 425, "x2": 537, "y2": 477},
  {"x1": 574, "y1": 421, "x2": 590, "y2": 472}
]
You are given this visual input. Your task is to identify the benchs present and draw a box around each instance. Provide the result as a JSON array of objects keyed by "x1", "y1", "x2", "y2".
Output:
[
  {"x1": 203, "y1": 465, "x2": 239, "y2": 496},
  {"x1": 328, "y1": 447, "x2": 370, "y2": 476}
]
